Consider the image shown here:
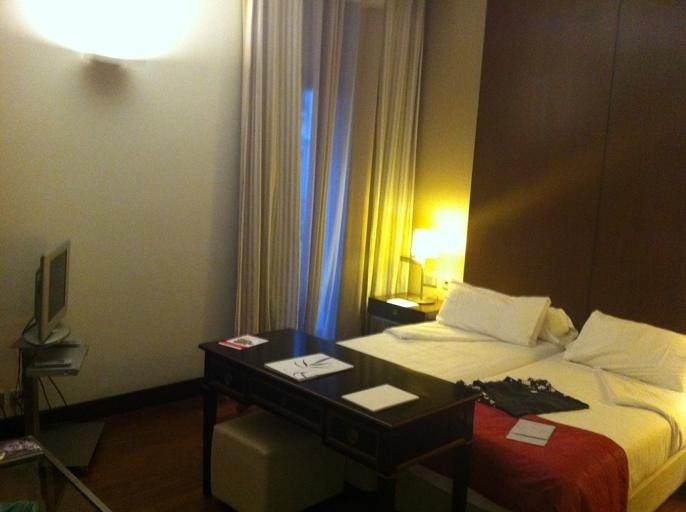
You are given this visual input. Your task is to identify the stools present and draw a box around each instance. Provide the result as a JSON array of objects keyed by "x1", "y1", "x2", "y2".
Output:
[{"x1": 210, "y1": 413, "x2": 346, "y2": 512}]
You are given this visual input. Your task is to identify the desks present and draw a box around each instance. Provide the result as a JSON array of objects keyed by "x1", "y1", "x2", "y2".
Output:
[{"x1": 196, "y1": 328, "x2": 482, "y2": 512}]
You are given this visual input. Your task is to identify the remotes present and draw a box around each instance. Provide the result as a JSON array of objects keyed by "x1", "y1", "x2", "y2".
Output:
[
  {"x1": 60, "y1": 340, "x2": 80, "y2": 347},
  {"x1": 33, "y1": 358, "x2": 72, "y2": 369}
]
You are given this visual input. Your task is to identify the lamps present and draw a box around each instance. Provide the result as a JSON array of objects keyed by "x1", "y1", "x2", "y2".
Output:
[{"x1": 400, "y1": 228, "x2": 439, "y2": 305}]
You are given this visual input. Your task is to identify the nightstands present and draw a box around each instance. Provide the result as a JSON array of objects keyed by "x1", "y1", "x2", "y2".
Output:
[{"x1": 367, "y1": 293, "x2": 443, "y2": 332}]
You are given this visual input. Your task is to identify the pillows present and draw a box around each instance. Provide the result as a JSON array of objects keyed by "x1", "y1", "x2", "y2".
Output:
[
  {"x1": 565, "y1": 309, "x2": 686, "y2": 394},
  {"x1": 435, "y1": 282, "x2": 550, "y2": 347}
]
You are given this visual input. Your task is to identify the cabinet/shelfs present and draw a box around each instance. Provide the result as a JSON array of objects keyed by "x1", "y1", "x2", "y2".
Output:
[{"x1": 13, "y1": 339, "x2": 106, "y2": 471}]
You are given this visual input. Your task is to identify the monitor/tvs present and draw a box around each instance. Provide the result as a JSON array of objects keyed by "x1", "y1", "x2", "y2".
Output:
[{"x1": 32, "y1": 237, "x2": 72, "y2": 342}]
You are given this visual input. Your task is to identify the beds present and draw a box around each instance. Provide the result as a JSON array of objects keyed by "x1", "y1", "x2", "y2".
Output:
[
  {"x1": 336, "y1": 280, "x2": 581, "y2": 387},
  {"x1": 410, "y1": 310, "x2": 686, "y2": 512}
]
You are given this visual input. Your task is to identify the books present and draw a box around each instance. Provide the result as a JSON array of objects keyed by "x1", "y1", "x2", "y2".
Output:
[
  {"x1": 0, "y1": 437, "x2": 44, "y2": 467},
  {"x1": 265, "y1": 352, "x2": 355, "y2": 381},
  {"x1": 218, "y1": 334, "x2": 269, "y2": 350},
  {"x1": 341, "y1": 384, "x2": 419, "y2": 413}
]
